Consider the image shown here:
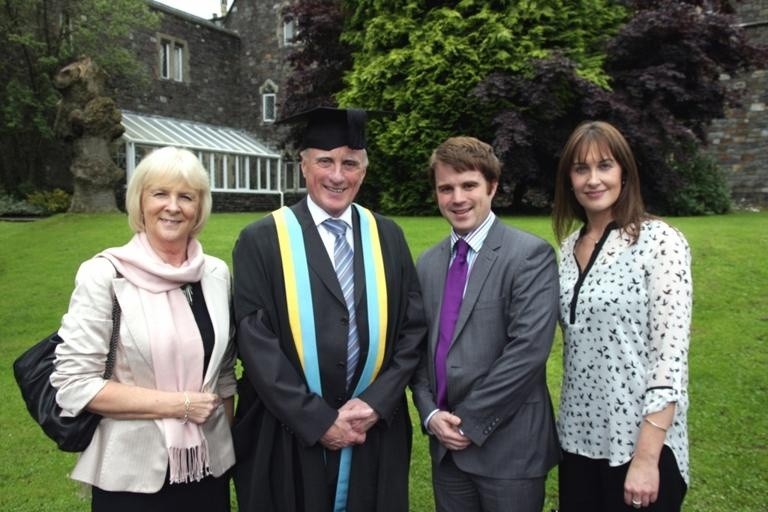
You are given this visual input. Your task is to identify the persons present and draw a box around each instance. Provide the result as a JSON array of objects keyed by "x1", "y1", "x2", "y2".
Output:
[
  {"x1": 553, "y1": 121, "x2": 691, "y2": 511},
  {"x1": 15, "y1": 147, "x2": 240, "y2": 512},
  {"x1": 228, "y1": 107, "x2": 430, "y2": 512},
  {"x1": 407, "y1": 136, "x2": 565, "y2": 511}
]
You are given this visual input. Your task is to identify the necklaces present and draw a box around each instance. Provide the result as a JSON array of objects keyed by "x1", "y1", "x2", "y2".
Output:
[{"x1": 586, "y1": 236, "x2": 599, "y2": 247}]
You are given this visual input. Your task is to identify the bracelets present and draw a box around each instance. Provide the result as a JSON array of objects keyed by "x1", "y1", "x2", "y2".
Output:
[
  {"x1": 644, "y1": 418, "x2": 667, "y2": 432},
  {"x1": 184, "y1": 391, "x2": 191, "y2": 422}
]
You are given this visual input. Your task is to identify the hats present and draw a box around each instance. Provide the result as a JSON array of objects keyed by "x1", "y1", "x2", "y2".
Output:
[{"x1": 271, "y1": 105, "x2": 401, "y2": 151}]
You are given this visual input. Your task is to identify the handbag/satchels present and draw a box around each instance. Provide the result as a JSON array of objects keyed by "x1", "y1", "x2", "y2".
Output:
[{"x1": 11, "y1": 269, "x2": 127, "y2": 452}]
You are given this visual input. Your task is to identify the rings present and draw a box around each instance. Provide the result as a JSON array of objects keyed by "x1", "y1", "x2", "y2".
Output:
[{"x1": 632, "y1": 498, "x2": 642, "y2": 505}]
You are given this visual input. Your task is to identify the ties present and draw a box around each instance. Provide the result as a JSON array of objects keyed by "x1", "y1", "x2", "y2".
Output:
[
  {"x1": 429, "y1": 239, "x2": 474, "y2": 413},
  {"x1": 320, "y1": 220, "x2": 365, "y2": 393}
]
